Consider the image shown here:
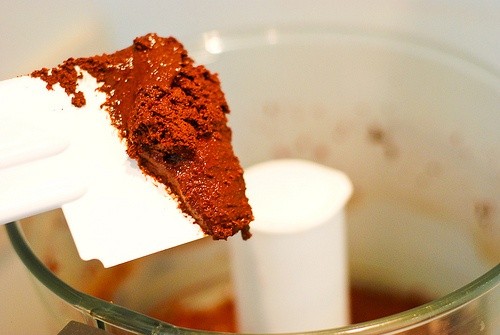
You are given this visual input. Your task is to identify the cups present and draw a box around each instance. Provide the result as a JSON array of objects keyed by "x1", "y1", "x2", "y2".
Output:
[{"x1": 5, "y1": 19, "x2": 500, "y2": 335}]
